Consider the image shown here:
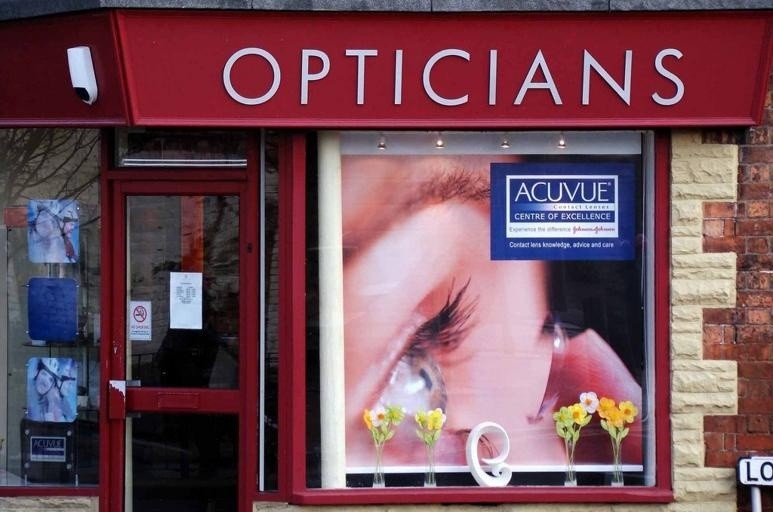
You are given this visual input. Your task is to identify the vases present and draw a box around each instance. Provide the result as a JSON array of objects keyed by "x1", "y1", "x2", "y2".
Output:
[
  {"x1": 563, "y1": 439, "x2": 623, "y2": 488},
  {"x1": 370, "y1": 439, "x2": 437, "y2": 488}
]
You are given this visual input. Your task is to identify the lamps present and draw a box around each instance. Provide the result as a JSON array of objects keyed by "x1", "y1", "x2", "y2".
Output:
[{"x1": 377, "y1": 131, "x2": 566, "y2": 149}]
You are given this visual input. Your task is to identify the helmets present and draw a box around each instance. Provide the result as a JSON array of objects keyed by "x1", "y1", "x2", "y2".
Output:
[
  {"x1": 37, "y1": 201, "x2": 79, "y2": 221},
  {"x1": 40, "y1": 358, "x2": 77, "y2": 381}
]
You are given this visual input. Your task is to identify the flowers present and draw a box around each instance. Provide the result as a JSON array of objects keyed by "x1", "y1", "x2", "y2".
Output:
[
  {"x1": 552, "y1": 391, "x2": 637, "y2": 484},
  {"x1": 362, "y1": 404, "x2": 446, "y2": 484}
]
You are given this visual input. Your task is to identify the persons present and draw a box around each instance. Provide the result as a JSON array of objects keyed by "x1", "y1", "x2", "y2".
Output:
[
  {"x1": 338, "y1": 156, "x2": 642, "y2": 472},
  {"x1": 46, "y1": 358, "x2": 75, "y2": 419},
  {"x1": 26, "y1": 357, "x2": 61, "y2": 421},
  {"x1": 27, "y1": 200, "x2": 59, "y2": 262},
  {"x1": 50, "y1": 207, "x2": 77, "y2": 263}
]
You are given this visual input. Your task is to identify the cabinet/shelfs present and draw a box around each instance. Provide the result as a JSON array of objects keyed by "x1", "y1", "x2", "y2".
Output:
[{"x1": 20, "y1": 228, "x2": 99, "y2": 487}]
